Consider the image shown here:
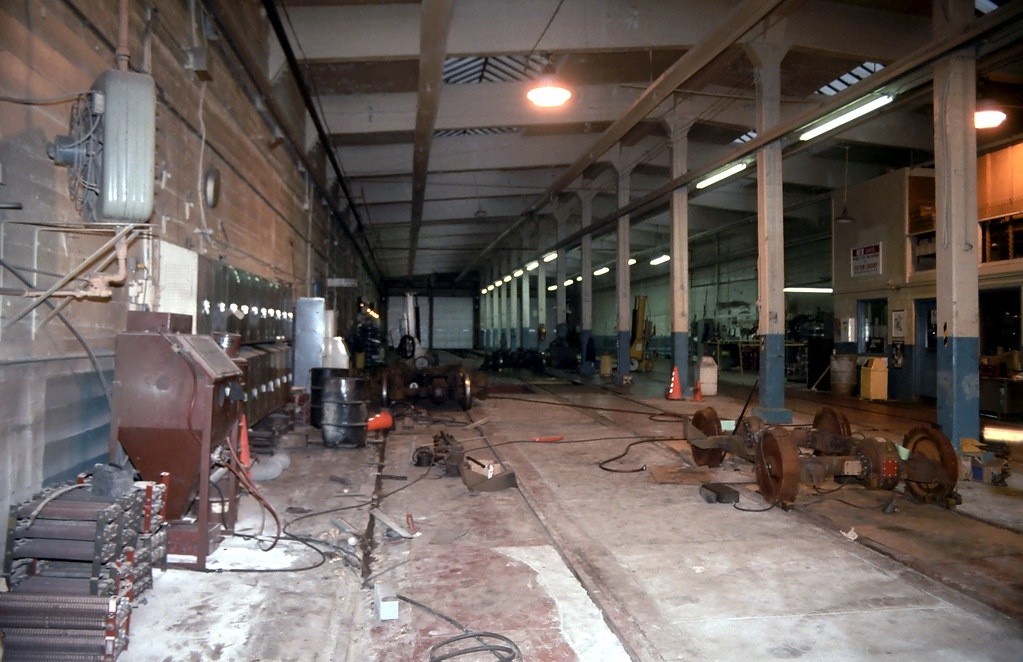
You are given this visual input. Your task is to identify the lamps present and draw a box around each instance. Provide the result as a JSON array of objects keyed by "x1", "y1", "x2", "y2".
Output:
[
  {"x1": 694, "y1": 157, "x2": 754, "y2": 191},
  {"x1": 974, "y1": 79, "x2": 1008, "y2": 129},
  {"x1": 481, "y1": 250, "x2": 671, "y2": 295},
  {"x1": 526, "y1": 59, "x2": 572, "y2": 108},
  {"x1": 793, "y1": 85, "x2": 896, "y2": 143}
]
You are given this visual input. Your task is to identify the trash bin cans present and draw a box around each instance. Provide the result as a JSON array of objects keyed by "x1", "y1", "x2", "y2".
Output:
[{"x1": 861, "y1": 357, "x2": 888, "y2": 402}]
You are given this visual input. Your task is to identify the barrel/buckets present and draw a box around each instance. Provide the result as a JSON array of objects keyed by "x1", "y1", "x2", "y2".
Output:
[
  {"x1": 321, "y1": 376, "x2": 368, "y2": 449},
  {"x1": 213, "y1": 332, "x2": 242, "y2": 359}
]
